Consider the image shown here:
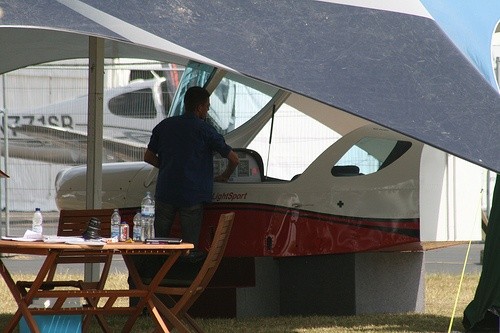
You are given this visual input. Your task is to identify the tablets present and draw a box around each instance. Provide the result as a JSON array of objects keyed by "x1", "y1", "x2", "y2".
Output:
[{"x1": 145, "y1": 238, "x2": 183, "y2": 244}]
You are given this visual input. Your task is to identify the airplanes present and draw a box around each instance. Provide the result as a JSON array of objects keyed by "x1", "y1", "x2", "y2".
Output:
[{"x1": 0, "y1": 68, "x2": 235, "y2": 166}]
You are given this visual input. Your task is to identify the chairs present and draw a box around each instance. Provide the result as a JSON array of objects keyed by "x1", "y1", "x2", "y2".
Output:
[
  {"x1": 16, "y1": 207, "x2": 141, "y2": 333},
  {"x1": 146, "y1": 211, "x2": 234, "y2": 333}
]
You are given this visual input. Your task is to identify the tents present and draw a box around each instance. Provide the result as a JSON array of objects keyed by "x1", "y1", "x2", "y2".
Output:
[{"x1": 0, "y1": 0, "x2": 500, "y2": 333}]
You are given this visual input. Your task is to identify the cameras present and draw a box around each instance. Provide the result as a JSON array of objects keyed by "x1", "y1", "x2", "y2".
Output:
[{"x1": 83, "y1": 217, "x2": 101, "y2": 240}]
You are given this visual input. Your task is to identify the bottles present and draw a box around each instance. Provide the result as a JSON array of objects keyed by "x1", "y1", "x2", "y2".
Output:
[
  {"x1": 32, "y1": 207, "x2": 43, "y2": 239},
  {"x1": 140, "y1": 191, "x2": 156, "y2": 241},
  {"x1": 132, "y1": 208, "x2": 143, "y2": 241},
  {"x1": 111, "y1": 208, "x2": 121, "y2": 242}
]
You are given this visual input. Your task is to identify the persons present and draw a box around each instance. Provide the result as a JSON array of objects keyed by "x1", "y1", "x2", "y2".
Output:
[{"x1": 144, "y1": 86, "x2": 238, "y2": 250}]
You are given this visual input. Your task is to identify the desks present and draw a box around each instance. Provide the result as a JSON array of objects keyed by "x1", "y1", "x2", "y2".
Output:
[{"x1": 0, "y1": 236, "x2": 193, "y2": 333}]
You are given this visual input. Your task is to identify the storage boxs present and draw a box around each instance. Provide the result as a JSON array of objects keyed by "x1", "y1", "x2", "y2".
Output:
[{"x1": 18, "y1": 298, "x2": 82, "y2": 333}]
